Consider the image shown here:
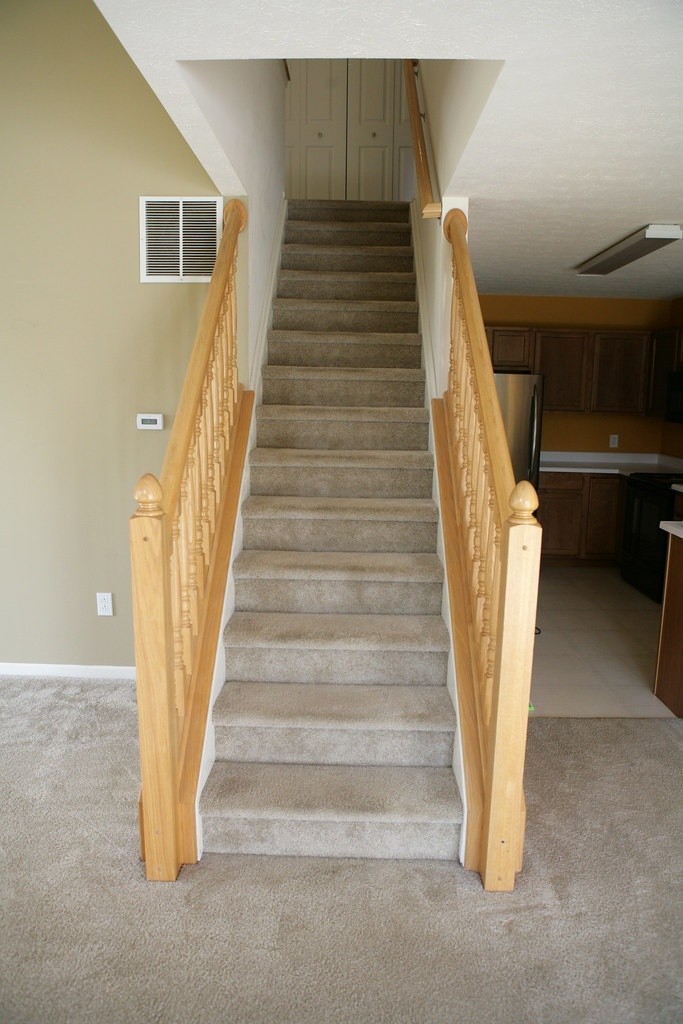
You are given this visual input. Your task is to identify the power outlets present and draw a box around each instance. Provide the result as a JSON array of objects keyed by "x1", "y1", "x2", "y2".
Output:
[
  {"x1": 96, "y1": 592, "x2": 114, "y2": 617},
  {"x1": 609, "y1": 434, "x2": 618, "y2": 448}
]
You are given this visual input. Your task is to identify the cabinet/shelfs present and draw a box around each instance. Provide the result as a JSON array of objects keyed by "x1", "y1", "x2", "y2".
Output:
[
  {"x1": 537, "y1": 472, "x2": 625, "y2": 561},
  {"x1": 481, "y1": 323, "x2": 683, "y2": 419}
]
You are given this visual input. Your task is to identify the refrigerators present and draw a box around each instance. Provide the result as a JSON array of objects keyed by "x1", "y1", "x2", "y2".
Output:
[{"x1": 486, "y1": 369, "x2": 543, "y2": 498}]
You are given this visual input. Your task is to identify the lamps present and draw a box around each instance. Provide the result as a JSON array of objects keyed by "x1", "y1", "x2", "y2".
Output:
[{"x1": 576, "y1": 224, "x2": 682, "y2": 278}]
